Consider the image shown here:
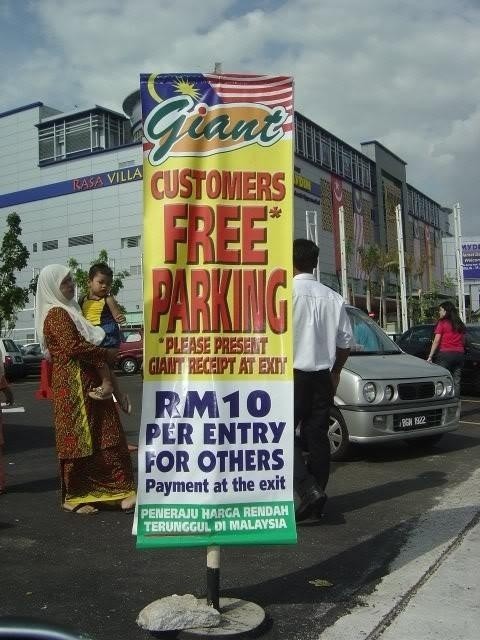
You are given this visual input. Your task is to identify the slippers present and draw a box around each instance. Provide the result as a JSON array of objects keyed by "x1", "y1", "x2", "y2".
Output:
[
  {"x1": 63, "y1": 503, "x2": 100, "y2": 516},
  {"x1": 88, "y1": 385, "x2": 114, "y2": 401},
  {"x1": 120, "y1": 390, "x2": 132, "y2": 416}
]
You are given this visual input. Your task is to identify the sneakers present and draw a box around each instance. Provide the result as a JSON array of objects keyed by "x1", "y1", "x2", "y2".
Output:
[{"x1": 124, "y1": 504, "x2": 136, "y2": 514}]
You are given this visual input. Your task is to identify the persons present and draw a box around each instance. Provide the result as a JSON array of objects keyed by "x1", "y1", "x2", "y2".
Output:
[
  {"x1": 426, "y1": 300, "x2": 470, "y2": 398},
  {"x1": 34, "y1": 264, "x2": 138, "y2": 517},
  {"x1": 293, "y1": 238, "x2": 354, "y2": 523},
  {"x1": 79, "y1": 262, "x2": 133, "y2": 416},
  {"x1": 0, "y1": 349, "x2": 16, "y2": 491}
]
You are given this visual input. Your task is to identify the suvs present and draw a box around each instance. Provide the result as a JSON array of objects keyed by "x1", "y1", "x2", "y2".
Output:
[{"x1": 0, "y1": 327, "x2": 144, "y2": 380}]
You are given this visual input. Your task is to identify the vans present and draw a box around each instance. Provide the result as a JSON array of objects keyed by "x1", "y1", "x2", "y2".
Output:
[{"x1": 327, "y1": 300, "x2": 467, "y2": 460}]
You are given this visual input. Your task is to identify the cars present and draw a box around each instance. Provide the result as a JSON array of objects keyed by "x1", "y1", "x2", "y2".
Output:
[{"x1": 389, "y1": 318, "x2": 480, "y2": 393}]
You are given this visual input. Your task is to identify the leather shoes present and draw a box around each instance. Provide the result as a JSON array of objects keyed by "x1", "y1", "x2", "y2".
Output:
[{"x1": 294, "y1": 482, "x2": 328, "y2": 522}]
